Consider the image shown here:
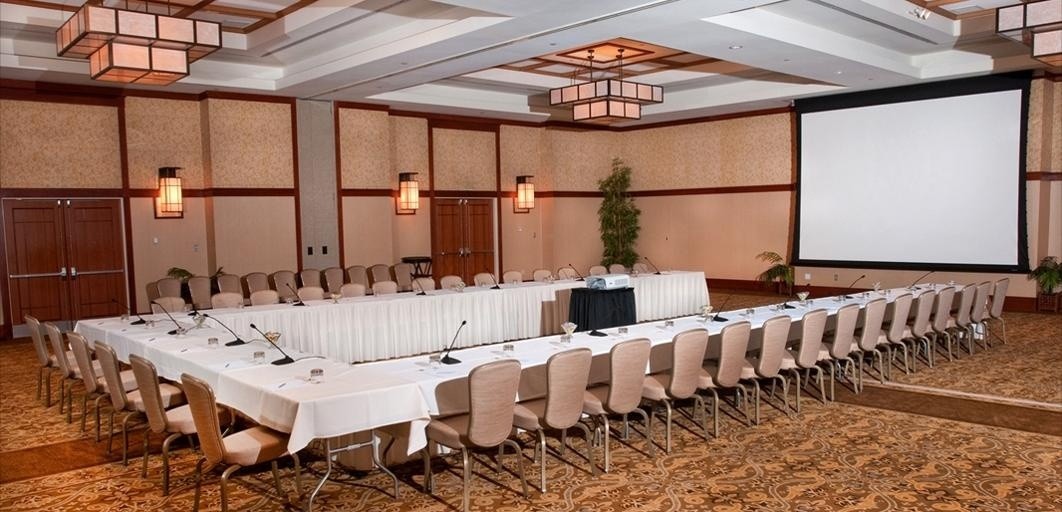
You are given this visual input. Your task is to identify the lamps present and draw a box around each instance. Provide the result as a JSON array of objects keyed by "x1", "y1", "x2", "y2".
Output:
[
  {"x1": 992, "y1": 0, "x2": 1062, "y2": 70},
  {"x1": 546, "y1": 43, "x2": 667, "y2": 129},
  {"x1": 907, "y1": 5, "x2": 931, "y2": 21},
  {"x1": 149, "y1": 163, "x2": 187, "y2": 221},
  {"x1": 389, "y1": 171, "x2": 421, "y2": 215},
  {"x1": 53, "y1": 1, "x2": 226, "y2": 88},
  {"x1": 512, "y1": 174, "x2": 537, "y2": 215}
]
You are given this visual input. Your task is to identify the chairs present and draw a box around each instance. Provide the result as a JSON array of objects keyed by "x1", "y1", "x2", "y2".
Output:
[
  {"x1": 559, "y1": 340, "x2": 655, "y2": 474},
  {"x1": 423, "y1": 360, "x2": 530, "y2": 510},
  {"x1": 498, "y1": 347, "x2": 597, "y2": 494},
  {"x1": 129, "y1": 354, "x2": 228, "y2": 497},
  {"x1": 626, "y1": 328, "x2": 710, "y2": 452},
  {"x1": 181, "y1": 372, "x2": 302, "y2": 510},
  {"x1": 145, "y1": 259, "x2": 659, "y2": 310},
  {"x1": 94, "y1": 340, "x2": 180, "y2": 451},
  {"x1": 23, "y1": 317, "x2": 138, "y2": 434}
]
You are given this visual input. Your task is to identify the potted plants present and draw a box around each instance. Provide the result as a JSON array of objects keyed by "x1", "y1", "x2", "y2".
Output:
[
  {"x1": 1025, "y1": 252, "x2": 1061, "y2": 316},
  {"x1": 751, "y1": 249, "x2": 796, "y2": 300},
  {"x1": 593, "y1": 153, "x2": 642, "y2": 276}
]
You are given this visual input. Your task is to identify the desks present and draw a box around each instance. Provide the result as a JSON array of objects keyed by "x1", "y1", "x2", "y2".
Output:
[
  {"x1": 364, "y1": 286, "x2": 996, "y2": 475},
  {"x1": 155, "y1": 272, "x2": 711, "y2": 363},
  {"x1": 75, "y1": 319, "x2": 433, "y2": 508}
]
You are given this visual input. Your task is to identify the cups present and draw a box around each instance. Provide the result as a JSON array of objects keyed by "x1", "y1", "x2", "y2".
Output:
[
  {"x1": 181, "y1": 290, "x2": 381, "y2": 316},
  {"x1": 832, "y1": 279, "x2": 957, "y2": 305},
  {"x1": 700, "y1": 305, "x2": 714, "y2": 324},
  {"x1": 121, "y1": 314, "x2": 130, "y2": 323},
  {"x1": 665, "y1": 321, "x2": 674, "y2": 331},
  {"x1": 253, "y1": 352, "x2": 266, "y2": 364},
  {"x1": 796, "y1": 291, "x2": 814, "y2": 308},
  {"x1": 175, "y1": 322, "x2": 186, "y2": 336},
  {"x1": 559, "y1": 321, "x2": 578, "y2": 348},
  {"x1": 311, "y1": 369, "x2": 324, "y2": 383},
  {"x1": 617, "y1": 327, "x2": 628, "y2": 338},
  {"x1": 776, "y1": 303, "x2": 784, "y2": 314},
  {"x1": 429, "y1": 355, "x2": 442, "y2": 371},
  {"x1": 145, "y1": 319, "x2": 155, "y2": 330},
  {"x1": 746, "y1": 308, "x2": 755, "y2": 321},
  {"x1": 207, "y1": 338, "x2": 219, "y2": 349}
]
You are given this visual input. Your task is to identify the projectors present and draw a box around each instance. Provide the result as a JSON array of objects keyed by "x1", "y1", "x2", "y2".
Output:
[{"x1": 586, "y1": 272, "x2": 630, "y2": 291}]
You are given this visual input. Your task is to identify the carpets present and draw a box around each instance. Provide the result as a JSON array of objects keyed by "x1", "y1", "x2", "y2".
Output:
[
  {"x1": 0, "y1": 335, "x2": 173, "y2": 457},
  {"x1": 0, "y1": 382, "x2": 1062, "y2": 512},
  {"x1": 703, "y1": 285, "x2": 1061, "y2": 417}
]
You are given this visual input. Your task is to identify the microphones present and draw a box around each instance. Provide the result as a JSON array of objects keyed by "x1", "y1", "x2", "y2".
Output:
[
  {"x1": 568, "y1": 263, "x2": 585, "y2": 281},
  {"x1": 714, "y1": 288, "x2": 736, "y2": 322},
  {"x1": 202, "y1": 313, "x2": 245, "y2": 348},
  {"x1": 441, "y1": 320, "x2": 466, "y2": 364},
  {"x1": 844, "y1": 273, "x2": 865, "y2": 299},
  {"x1": 643, "y1": 256, "x2": 663, "y2": 275},
  {"x1": 408, "y1": 270, "x2": 428, "y2": 296},
  {"x1": 907, "y1": 269, "x2": 939, "y2": 289},
  {"x1": 148, "y1": 300, "x2": 185, "y2": 335},
  {"x1": 285, "y1": 283, "x2": 305, "y2": 307},
  {"x1": 784, "y1": 282, "x2": 811, "y2": 309},
  {"x1": 484, "y1": 263, "x2": 503, "y2": 289},
  {"x1": 249, "y1": 323, "x2": 295, "y2": 366},
  {"x1": 589, "y1": 304, "x2": 620, "y2": 337},
  {"x1": 110, "y1": 298, "x2": 145, "y2": 326}
]
